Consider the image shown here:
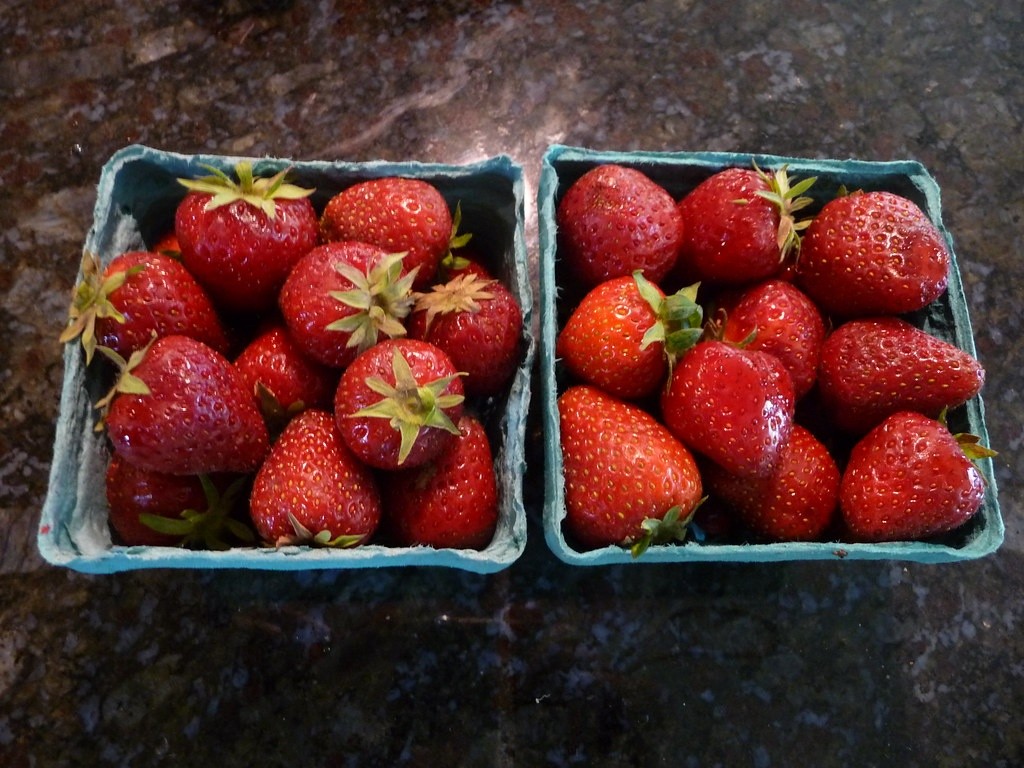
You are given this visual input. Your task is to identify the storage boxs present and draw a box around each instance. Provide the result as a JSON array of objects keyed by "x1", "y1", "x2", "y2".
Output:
[
  {"x1": 36, "y1": 143, "x2": 536, "y2": 578},
  {"x1": 540, "y1": 144, "x2": 1007, "y2": 567}
]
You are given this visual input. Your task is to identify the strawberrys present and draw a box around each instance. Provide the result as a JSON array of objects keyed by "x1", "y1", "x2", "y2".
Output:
[
  {"x1": 57, "y1": 160, "x2": 523, "y2": 553},
  {"x1": 554, "y1": 157, "x2": 1000, "y2": 560}
]
